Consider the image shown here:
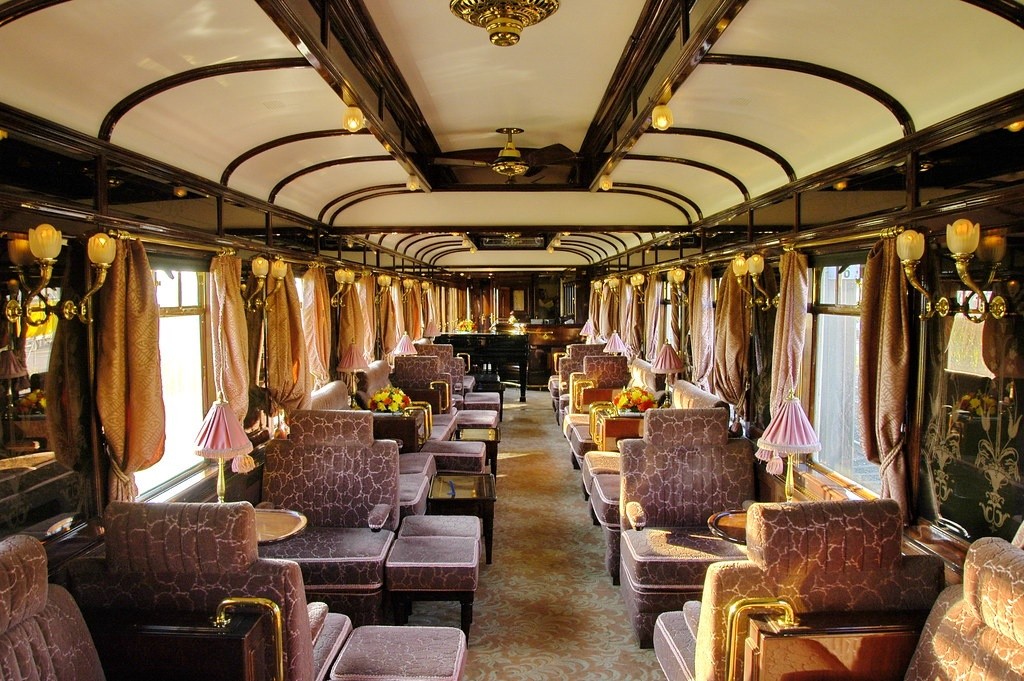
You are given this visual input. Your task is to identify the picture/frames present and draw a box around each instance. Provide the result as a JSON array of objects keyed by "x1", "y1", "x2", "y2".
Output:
[{"x1": 510, "y1": 286, "x2": 529, "y2": 315}]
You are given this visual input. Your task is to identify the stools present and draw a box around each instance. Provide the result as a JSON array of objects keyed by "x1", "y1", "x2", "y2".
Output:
[
  {"x1": 418, "y1": 439, "x2": 486, "y2": 474},
  {"x1": 328, "y1": 623, "x2": 469, "y2": 681},
  {"x1": 457, "y1": 409, "x2": 500, "y2": 429},
  {"x1": 382, "y1": 514, "x2": 482, "y2": 650},
  {"x1": 465, "y1": 392, "x2": 501, "y2": 411}
]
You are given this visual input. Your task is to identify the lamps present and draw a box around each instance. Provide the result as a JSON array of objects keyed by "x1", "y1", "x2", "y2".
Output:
[
  {"x1": 451, "y1": 232, "x2": 477, "y2": 255},
  {"x1": 0, "y1": 345, "x2": 30, "y2": 446},
  {"x1": 547, "y1": 232, "x2": 571, "y2": 254},
  {"x1": 336, "y1": 338, "x2": 370, "y2": 409},
  {"x1": 423, "y1": 319, "x2": 442, "y2": 344},
  {"x1": 593, "y1": 102, "x2": 1005, "y2": 325},
  {"x1": 60, "y1": 232, "x2": 116, "y2": 324},
  {"x1": 193, "y1": 390, "x2": 255, "y2": 504},
  {"x1": 242, "y1": 103, "x2": 431, "y2": 313},
  {"x1": 7, "y1": 238, "x2": 50, "y2": 326},
  {"x1": 392, "y1": 331, "x2": 418, "y2": 357},
  {"x1": 602, "y1": 330, "x2": 627, "y2": 356},
  {"x1": 755, "y1": 388, "x2": 822, "y2": 502},
  {"x1": 651, "y1": 339, "x2": 689, "y2": 406},
  {"x1": 579, "y1": 318, "x2": 599, "y2": 343},
  {"x1": 3, "y1": 223, "x2": 64, "y2": 323}
]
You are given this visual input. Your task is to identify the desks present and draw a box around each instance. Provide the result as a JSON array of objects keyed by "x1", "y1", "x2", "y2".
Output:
[
  {"x1": 254, "y1": 508, "x2": 309, "y2": 546},
  {"x1": 705, "y1": 505, "x2": 748, "y2": 549},
  {"x1": 428, "y1": 473, "x2": 498, "y2": 567},
  {"x1": 473, "y1": 383, "x2": 506, "y2": 422},
  {"x1": 434, "y1": 332, "x2": 529, "y2": 403},
  {"x1": 455, "y1": 427, "x2": 499, "y2": 485}
]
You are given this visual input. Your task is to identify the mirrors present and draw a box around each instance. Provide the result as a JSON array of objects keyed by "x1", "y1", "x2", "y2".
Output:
[
  {"x1": 0, "y1": 235, "x2": 98, "y2": 544},
  {"x1": 908, "y1": 224, "x2": 1024, "y2": 547}
]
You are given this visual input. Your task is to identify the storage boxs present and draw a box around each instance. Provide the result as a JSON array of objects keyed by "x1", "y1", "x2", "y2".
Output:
[{"x1": 531, "y1": 319, "x2": 543, "y2": 325}]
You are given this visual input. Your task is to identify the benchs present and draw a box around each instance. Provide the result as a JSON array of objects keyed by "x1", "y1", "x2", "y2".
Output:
[
  {"x1": 545, "y1": 342, "x2": 1024, "y2": 681},
  {"x1": 0, "y1": 342, "x2": 475, "y2": 681}
]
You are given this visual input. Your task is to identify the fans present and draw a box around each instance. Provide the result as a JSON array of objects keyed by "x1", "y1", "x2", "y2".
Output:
[{"x1": 430, "y1": 125, "x2": 586, "y2": 182}]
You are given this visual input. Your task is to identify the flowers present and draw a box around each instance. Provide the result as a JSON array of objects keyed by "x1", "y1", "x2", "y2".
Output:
[
  {"x1": 457, "y1": 318, "x2": 477, "y2": 332},
  {"x1": 369, "y1": 383, "x2": 413, "y2": 415},
  {"x1": 613, "y1": 385, "x2": 657, "y2": 414},
  {"x1": 18, "y1": 387, "x2": 48, "y2": 415}
]
